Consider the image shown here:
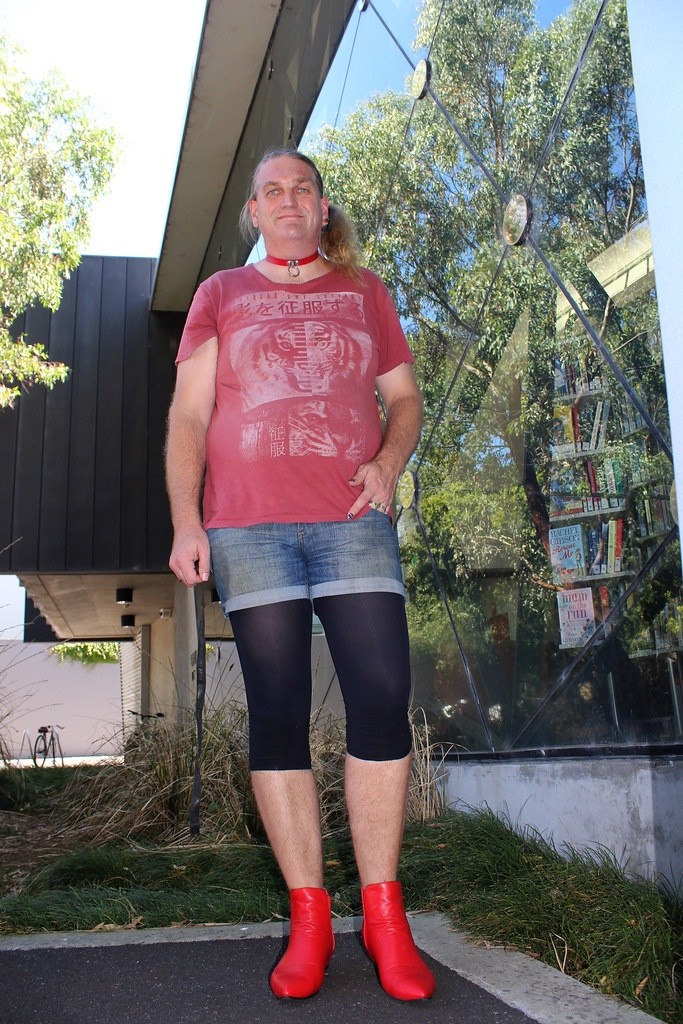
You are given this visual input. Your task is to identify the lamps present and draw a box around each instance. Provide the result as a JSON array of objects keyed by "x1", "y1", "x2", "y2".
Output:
[
  {"x1": 116, "y1": 588, "x2": 133, "y2": 608},
  {"x1": 121, "y1": 615, "x2": 136, "y2": 628}
]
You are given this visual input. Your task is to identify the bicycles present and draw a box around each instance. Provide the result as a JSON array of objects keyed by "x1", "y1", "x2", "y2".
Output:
[{"x1": 32, "y1": 724, "x2": 65, "y2": 768}]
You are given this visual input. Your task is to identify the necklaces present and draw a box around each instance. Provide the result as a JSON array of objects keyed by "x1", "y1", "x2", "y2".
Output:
[{"x1": 266, "y1": 250, "x2": 320, "y2": 277}]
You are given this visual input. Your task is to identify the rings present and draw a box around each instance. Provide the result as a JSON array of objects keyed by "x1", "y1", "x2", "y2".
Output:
[
  {"x1": 378, "y1": 504, "x2": 386, "y2": 512},
  {"x1": 178, "y1": 579, "x2": 182, "y2": 582},
  {"x1": 369, "y1": 501, "x2": 378, "y2": 506}
]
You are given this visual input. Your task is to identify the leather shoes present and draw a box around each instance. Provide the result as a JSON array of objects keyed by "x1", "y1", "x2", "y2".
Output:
[
  {"x1": 270, "y1": 887, "x2": 334, "y2": 1001},
  {"x1": 360, "y1": 882, "x2": 435, "y2": 1003}
]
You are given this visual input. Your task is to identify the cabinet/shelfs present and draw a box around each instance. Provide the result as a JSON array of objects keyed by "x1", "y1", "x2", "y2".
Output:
[{"x1": 550, "y1": 386, "x2": 680, "y2": 737}]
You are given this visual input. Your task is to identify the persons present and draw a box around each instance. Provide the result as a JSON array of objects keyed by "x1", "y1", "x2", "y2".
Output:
[{"x1": 164, "y1": 148, "x2": 436, "y2": 1001}]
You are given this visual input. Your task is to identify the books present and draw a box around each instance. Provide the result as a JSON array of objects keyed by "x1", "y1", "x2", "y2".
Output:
[{"x1": 547, "y1": 344, "x2": 627, "y2": 644}]
